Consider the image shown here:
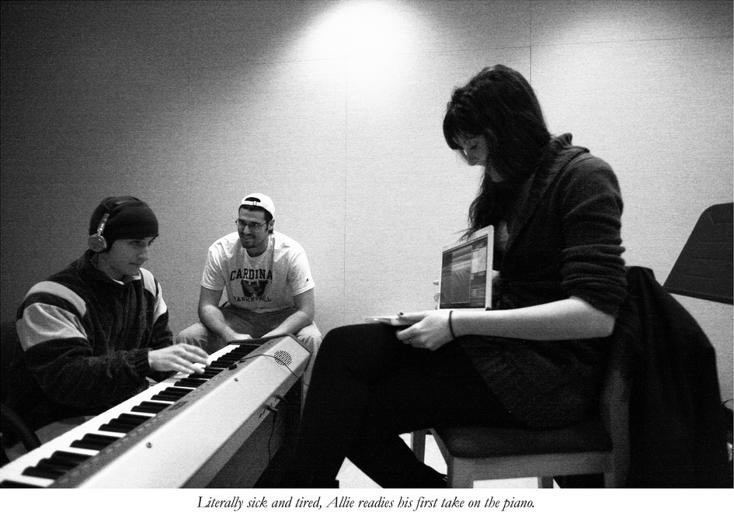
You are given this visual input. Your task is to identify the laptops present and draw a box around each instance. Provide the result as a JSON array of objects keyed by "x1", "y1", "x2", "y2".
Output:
[{"x1": 364, "y1": 224, "x2": 495, "y2": 326}]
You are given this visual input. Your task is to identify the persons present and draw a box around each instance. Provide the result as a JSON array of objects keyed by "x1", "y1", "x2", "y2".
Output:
[
  {"x1": 287, "y1": 66, "x2": 629, "y2": 488},
  {"x1": 175, "y1": 193, "x2": 323, "y2": 428},
  {"x1": 0, "y1": 196, "x2": 209, "y2": 465}
]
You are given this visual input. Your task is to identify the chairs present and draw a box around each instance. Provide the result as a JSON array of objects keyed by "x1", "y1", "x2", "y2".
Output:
[
  {"x1": 0, "y1": 320, "x2": 62, "y2": 452},
  {"x1": 409, "y1": 266, "x2": 652, "y2": 488}
]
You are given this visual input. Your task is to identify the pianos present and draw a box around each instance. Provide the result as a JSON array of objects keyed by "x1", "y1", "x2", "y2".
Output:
[{"x1": 1, "y1": 332, "x2": 313, "y2": 488}]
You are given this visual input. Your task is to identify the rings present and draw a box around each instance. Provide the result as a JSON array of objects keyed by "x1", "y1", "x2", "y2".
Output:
[{"x1": 409, "y1": 339, "x2": 412, "y2": 345}]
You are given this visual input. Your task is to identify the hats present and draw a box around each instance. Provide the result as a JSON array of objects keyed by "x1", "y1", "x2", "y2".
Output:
[
  {"x1": 239, "y1": 192, "x2": 275, "y2": 219},
  {"x1": 89, "y1": 195, "x2": 159, "y2": 253}
]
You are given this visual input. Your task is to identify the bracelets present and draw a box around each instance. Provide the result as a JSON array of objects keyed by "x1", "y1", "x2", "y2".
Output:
[{"x1": 448, "y1": 310, "x2": 458, "y2": 340}]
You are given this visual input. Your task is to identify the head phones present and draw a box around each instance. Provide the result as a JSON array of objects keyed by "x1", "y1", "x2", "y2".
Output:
[{"x1": 88, "y1": 195, "x2": 140, "y2": 253}]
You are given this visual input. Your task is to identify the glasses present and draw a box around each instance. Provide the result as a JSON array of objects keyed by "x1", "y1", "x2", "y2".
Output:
[{"x1": 235, "y1": 217, "x2": 270, "y2": 232}]
